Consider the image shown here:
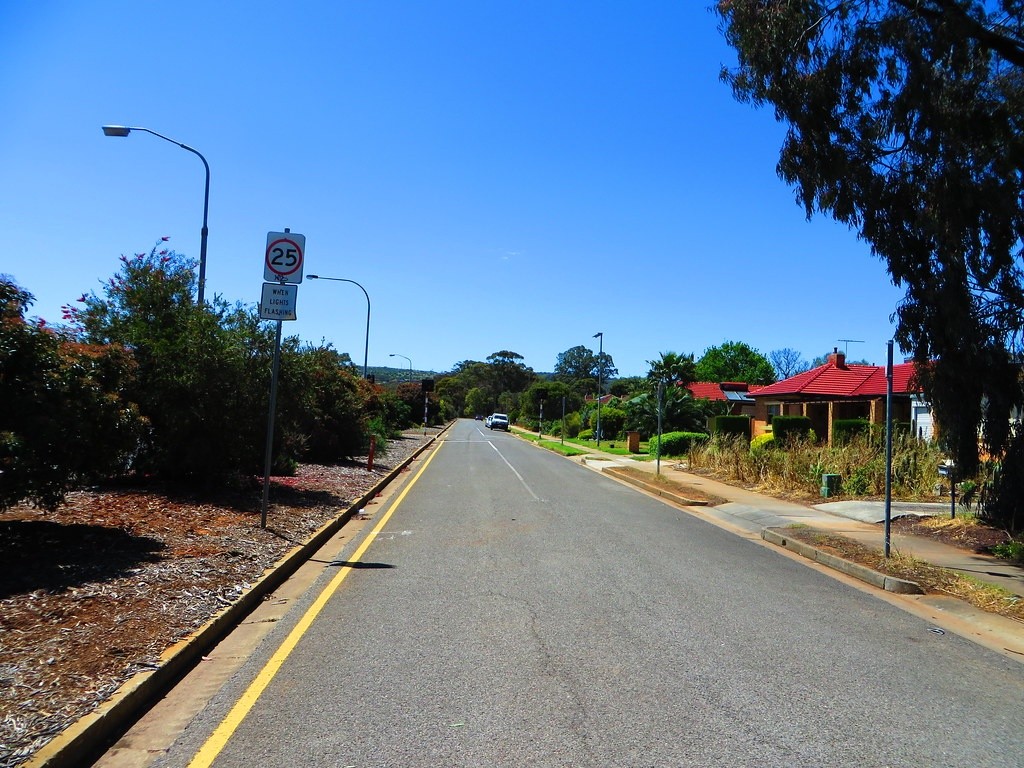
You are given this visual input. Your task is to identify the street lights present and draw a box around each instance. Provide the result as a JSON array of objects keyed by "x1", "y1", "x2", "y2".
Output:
[
  {"x1": 305, "y1": 274, "x2": 371, "y2": 380},
  {"x1": 593, "y1": 333, "x2": 602, "y2": 446},
  {"x1": 101, "y1": 124, "x2": 210, "y2": 310},
  {"x1": 388, "y1": 354, "x2": 412, "y2": 384}
]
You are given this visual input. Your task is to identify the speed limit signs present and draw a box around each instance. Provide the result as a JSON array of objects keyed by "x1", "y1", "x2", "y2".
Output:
[{"x1": 263, "y1": 229, "x2": 306, "y2": 285}]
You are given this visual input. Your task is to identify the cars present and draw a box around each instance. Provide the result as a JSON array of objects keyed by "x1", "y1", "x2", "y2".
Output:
[
  {"x1": 475, "y1": 415, "x2": 483, "y2": 421},
  {"x1": 486, "y1": 416, "x2": 493, "y2": 428},
  {"x1": 490, "y1": 413, "x2": 508, "y2": 431}
]
